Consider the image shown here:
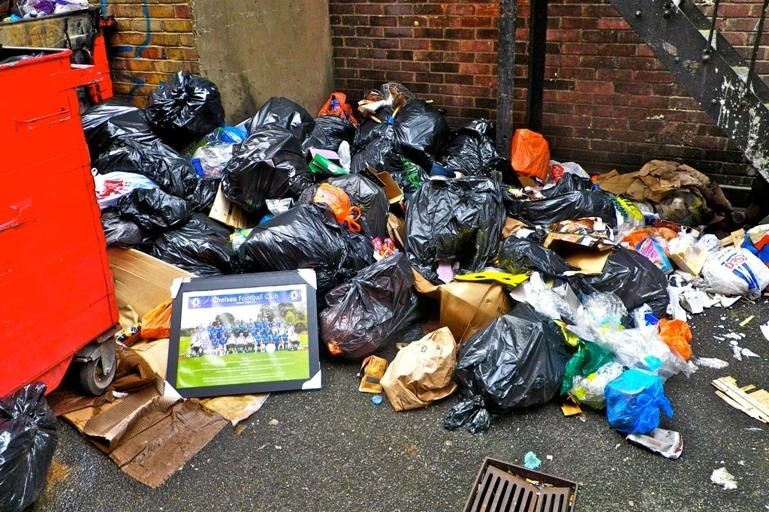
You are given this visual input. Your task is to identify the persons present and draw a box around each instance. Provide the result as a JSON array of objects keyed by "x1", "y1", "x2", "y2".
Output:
[{"x1": 185, "y1": 315, "x2": 301, "y2": 358}]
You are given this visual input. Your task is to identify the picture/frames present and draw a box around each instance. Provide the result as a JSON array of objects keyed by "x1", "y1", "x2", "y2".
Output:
[{"x1": 163, "y1": 268, "x2": 323, "y2": 400}]
